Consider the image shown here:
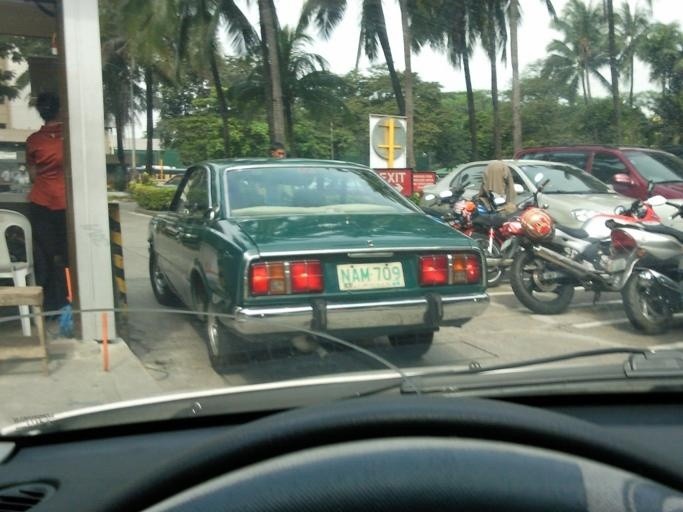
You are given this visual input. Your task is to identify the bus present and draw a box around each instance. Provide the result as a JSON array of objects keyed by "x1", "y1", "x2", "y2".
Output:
[{"x1": 126, "y1": 165, "x2": 187, "y2": 185}]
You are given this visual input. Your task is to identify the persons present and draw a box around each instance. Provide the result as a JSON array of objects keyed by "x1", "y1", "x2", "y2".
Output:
[
  {"x1": 23, "y1": 93, "x2": 69, "y2": 321},
  {"x1": 0, "y1": 44, "x2": 44, "y2": 127},
  {"x1": 3, "y1": 165, "x2": 30, "y2": 184},
  {"x1": 267, "y1": 141, "x2": 286, "y2": 158}
]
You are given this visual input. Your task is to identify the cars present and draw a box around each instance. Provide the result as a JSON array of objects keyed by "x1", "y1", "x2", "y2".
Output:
[
  {"x1": 425, "y1": 143, "x2": 682, "y2": 240},
  {"x1": 147, "y1": 155, "x2": 490, "y2": 374},
  {"x1": 156, "y1": 172, "x2": 186, "y2": 188}
]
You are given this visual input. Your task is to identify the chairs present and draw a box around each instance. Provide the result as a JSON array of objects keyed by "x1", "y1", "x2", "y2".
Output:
[{"x1": 0, "y1": 208, "x2": 39, "y2": 338}]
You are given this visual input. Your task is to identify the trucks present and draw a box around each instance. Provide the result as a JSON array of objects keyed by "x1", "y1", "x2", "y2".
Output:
[{"x1": 0, "y1": 128, "x2": 40, "y2": 219}]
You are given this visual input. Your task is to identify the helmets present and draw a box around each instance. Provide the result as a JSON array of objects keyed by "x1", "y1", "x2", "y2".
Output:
[{"x1": 518, "y1": 206, "x2": 556, "y2": 244}]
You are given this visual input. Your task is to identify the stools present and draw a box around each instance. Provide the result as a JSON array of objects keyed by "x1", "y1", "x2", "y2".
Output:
[{"x1": 0, "y1": 286, "x2": 52, "y2": 378}]
